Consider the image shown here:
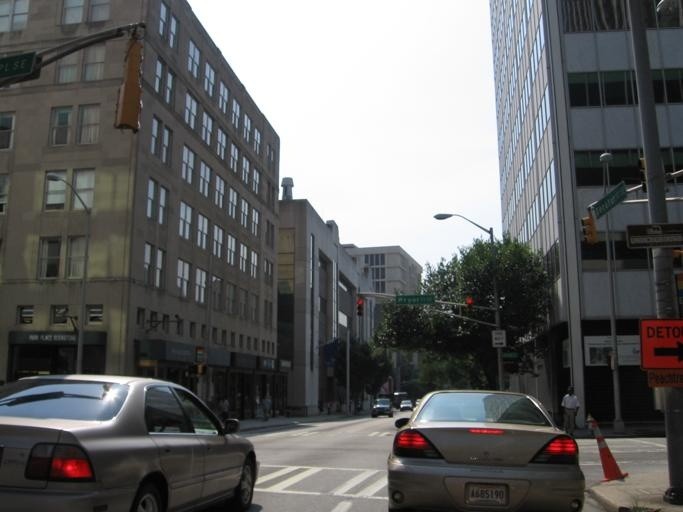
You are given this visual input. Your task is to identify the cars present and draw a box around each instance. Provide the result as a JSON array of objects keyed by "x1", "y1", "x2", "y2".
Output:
[
  {"x1": 372, "y1": 391, "x2": 416, "y2": 417},
  {"x1": 0, "y1": 374, "x2": 257, "y2": 512},
  {"x1": 388, "y1": 389, "x2": 585, "y2": 512}
]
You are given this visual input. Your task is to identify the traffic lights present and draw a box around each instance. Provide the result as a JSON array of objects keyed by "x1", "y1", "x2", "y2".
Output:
[
  {"x1": 466, "y1": 296, "x2": 473, "y2": 315},
  {"x1": 356, "y1": 294, "x2": 364, "y2": 317},
  {"x1": 581, "y1": 208, "x2": 600, "y2": 247}
]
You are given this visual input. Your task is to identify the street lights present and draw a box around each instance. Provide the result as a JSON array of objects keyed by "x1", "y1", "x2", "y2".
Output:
[
  {"x1": 597, "y1": 152, "x2": 626, "y2": 432},
  {"x1": 433, "y1": 213, "x2": 507, "y2": 390},
  {"x1": 43, "y1": 173, "x2": 92, "y2": 373}
]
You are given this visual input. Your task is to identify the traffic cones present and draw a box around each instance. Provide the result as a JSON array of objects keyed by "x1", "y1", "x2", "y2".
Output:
[{"x1": 590, "y1": 420, "x2": 629, "y2": 481}]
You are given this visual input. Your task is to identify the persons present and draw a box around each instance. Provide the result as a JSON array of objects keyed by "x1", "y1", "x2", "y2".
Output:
[
  {"x1": 263, "y1": 397, "x2": 274, "y2": 420},
  {"x1": 220, "y1": 396, "x2": 232, "y2": 426},
  {"x1": 560, "y1": 385, "x2": 581, "y2": 436}
]
